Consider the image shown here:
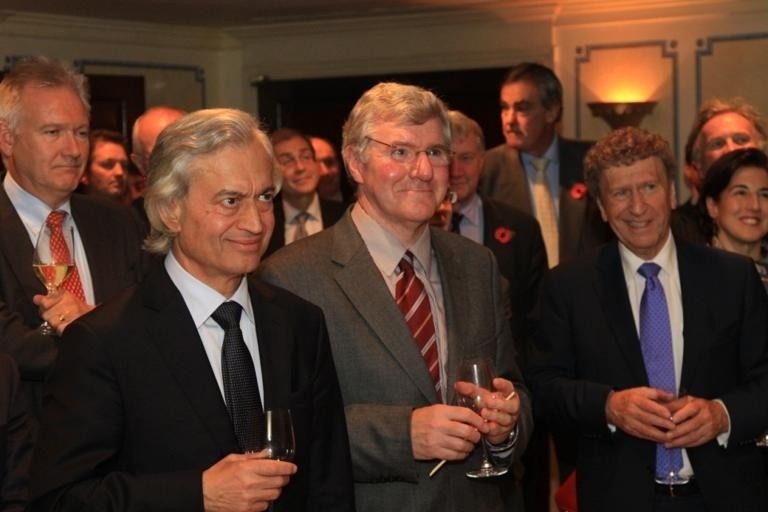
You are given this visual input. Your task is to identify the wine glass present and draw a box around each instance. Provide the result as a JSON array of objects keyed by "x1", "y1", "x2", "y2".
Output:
[
  {"x1": 31, "y1": 223, "x2": 77, "y2": 335},
  {"x1": 454, "y1": 358, "x2": 509, "y2": 478},
  {"x1": 246, "y1": 407, "x2": 297, "y2": 461},
  {"x1": 654, "y1": 386, "x2": 690, "y2": 488}
]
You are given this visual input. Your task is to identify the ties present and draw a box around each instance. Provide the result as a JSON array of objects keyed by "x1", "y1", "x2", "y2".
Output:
[
  {"x1": 41, "y1": 207, "x2": 91, "y2": 309},
  {"x1": 632, "y1": 260, "x2": 687, "y2": 483},
  {"x1": 292, "y1": 209, "x2": 314, "y2": 244},
  {"x1": 391, "y1": 244, "x2": 447, "y2": 408},
  {"x1": 526, "y1": 154, "x2": 563, "y2": 273},
  {"x1": 445, "y1": 206, "x2": 465, "y2": 243},
  {"x1": 210, "y1": 297, "x2": 271, "y2": 461}
]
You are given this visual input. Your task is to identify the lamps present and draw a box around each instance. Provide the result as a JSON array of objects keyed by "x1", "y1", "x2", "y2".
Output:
[{"x1": 585, "y1": 99, "x2": 658, "y2": 130}]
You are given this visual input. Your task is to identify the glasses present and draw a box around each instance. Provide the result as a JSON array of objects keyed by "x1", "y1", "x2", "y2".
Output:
[{"x1": 382, "y1": 140, "x2": 457, "y2": 168}]
